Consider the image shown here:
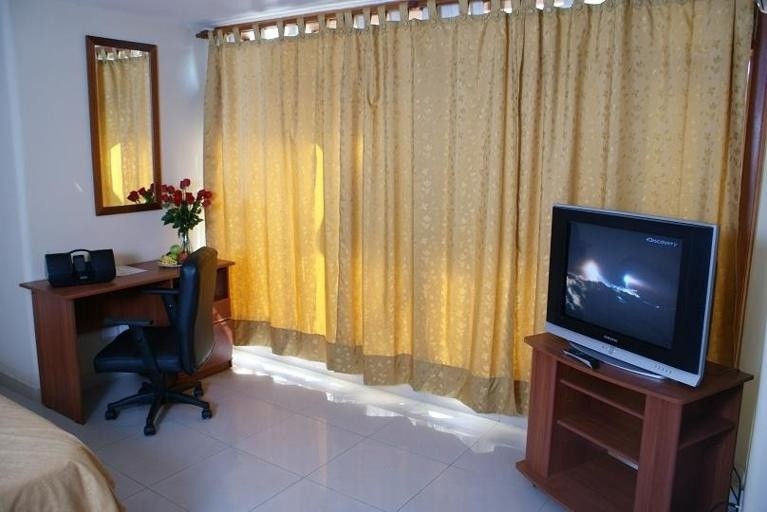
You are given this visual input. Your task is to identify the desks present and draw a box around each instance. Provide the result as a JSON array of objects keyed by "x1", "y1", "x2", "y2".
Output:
[{"x1": 17, "y1": 253, "x2": 235, "y2": 425}]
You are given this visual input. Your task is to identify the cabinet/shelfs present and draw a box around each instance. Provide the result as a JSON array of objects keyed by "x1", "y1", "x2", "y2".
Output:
[{"x1": 514, "y1": 332, "x2": 754, "y2": 512}]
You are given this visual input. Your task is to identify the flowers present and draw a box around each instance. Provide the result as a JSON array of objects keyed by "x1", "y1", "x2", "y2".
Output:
[
  {"x1": 156, "y1": 179, "x2": 212, "y2": 255},
  {"x1": 126, "y1": 183, "x2": 155, "y2": 205}
]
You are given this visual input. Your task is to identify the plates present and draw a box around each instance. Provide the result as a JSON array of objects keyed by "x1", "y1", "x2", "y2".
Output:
[{"x1": 157, "y1": 262, "x2": 183, "y2": 268}]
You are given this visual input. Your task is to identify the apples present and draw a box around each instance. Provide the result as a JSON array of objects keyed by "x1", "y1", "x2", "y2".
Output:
[{"x1": 168, "y1": 245, "x2": 188, "y2": 265}]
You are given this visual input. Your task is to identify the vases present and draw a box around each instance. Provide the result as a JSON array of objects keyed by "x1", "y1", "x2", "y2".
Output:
[{"x1": 179, "y1": 230, "x2": 192, "y2": 256}]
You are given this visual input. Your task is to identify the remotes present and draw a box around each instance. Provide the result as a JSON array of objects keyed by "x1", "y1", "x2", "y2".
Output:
[{"x1": 562, "y1": 347, "x2": 600, "y2": 369}]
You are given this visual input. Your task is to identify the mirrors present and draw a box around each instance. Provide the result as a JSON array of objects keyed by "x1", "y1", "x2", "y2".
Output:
[{"x1": 84, "y1": 35, "x2": 162, "y2": 217}]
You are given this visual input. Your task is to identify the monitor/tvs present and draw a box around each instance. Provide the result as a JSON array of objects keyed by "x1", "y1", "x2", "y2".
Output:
[{"x1": 544, "y1": 204, "x2": 720, "y2": 387}]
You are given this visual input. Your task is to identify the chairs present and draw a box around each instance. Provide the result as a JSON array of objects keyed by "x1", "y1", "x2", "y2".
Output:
[{"x1": 91, "y1": 246, "x2": 217, "y2": 436}]
